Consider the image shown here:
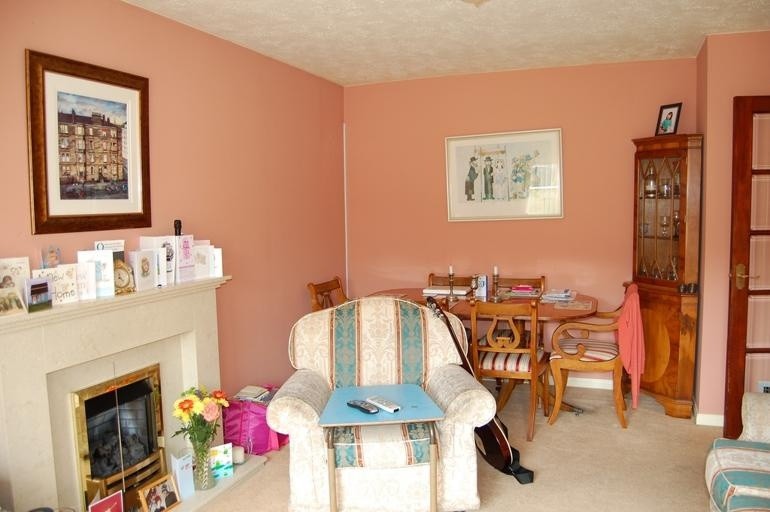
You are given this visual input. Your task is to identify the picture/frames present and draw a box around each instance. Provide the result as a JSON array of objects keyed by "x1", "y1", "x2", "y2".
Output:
[
  {"x1": 138, "y1": 474, "x2": 181, "y2": 512},
  {"x1": 444, "y1": 128, "x2": 564, "y2": 222},
  {"x1": 88, "y1": 490, "x2": 124, "y2": 511},
  {"x1": 655, "y1": 102, "x2": 682, "y2": 135},
  {"x1": 25, "y1": 50, "x2": 152, "y2": 235}
]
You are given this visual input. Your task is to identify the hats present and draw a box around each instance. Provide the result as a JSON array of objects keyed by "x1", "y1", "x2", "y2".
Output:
[
  {"x1": 484, "y1": 157, "x2": 493, "y2": 161},
  {"x1": 469, "y1": 156, "x2": 478, "y2": 163}
]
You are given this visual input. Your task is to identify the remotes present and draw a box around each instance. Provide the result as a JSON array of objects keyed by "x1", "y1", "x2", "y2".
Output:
[
  {"x1": 367, "y1": 396, "x2": 401, "y2": 414},
  {"x1": 347, "y1": 400, "x2": 379, "y2": 414}
]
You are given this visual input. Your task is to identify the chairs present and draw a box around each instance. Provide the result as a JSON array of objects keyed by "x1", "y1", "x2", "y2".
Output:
[
  {"x1": 547, "y1": 284, "x2": 646, "y2": 428},
  {"x1": 307, "y1": 276, "x2": 352, "y2": 312},
  {"x1": 705, "y1": 393, "x2": 769, "y2": 511},
  {"x1": 468, "y1": 298, "x2": 550, "y2": 441},
  {"x1": 428, "y1": 272, "x2": 474, "y2": 287},
  {"x1": 413, "y1": 298, "x2": 449, "y2": 313},
  {"x1": 496, "y1": 276, "x2": 546, "y2": 293},
  {"x1": 265, "y1": 297, "x2": 497, "y2": 512}
]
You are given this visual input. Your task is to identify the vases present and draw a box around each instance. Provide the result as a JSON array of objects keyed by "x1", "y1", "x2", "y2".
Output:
[{"x1": 184, "y1": 433, "x2": 216, "y2": 489}]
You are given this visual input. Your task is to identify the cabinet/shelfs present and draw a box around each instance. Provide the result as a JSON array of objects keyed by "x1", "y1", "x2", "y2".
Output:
[{"x1": 622, "y1": 134, "x2": 702, "y2": 419}]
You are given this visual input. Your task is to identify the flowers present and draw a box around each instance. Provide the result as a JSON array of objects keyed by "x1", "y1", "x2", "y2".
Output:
[{"x1": 171, "y1": 385, "x2": 230, "y2": 481}]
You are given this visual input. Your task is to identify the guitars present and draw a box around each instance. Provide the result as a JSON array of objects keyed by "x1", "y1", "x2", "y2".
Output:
[{"x1": 427, "y1": 296, "x2": 514, "y2": 470}]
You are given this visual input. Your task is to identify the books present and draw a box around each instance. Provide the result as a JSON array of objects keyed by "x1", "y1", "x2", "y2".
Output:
[
  {"x1": 504, "y1": 284, "x2": 593, "y2": 311},
  {"x1": 233, "y1": 385, "x2": 270, "y2": 402},
  {"x1": 422, "y1": 286, "x2": 473, "y2": 295}
]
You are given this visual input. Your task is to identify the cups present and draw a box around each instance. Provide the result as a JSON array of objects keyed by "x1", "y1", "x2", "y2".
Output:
[
  {"x1": 687, "y1": 284, "x2": 697, "y2": 293},
  {"x1": 677, "y1": 283, "x2": 687, "y2": 293}
]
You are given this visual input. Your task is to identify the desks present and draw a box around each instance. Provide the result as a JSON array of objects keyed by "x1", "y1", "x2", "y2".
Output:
[
  {"x1": 0, "y1": 273, "x2": 268, "y2": 512},
  {"x1": 365, "y1": 288, "x2": 597, "y2": 412},
  {"x1": 319, "y1": 384, "x2": 445, "y2": 512}
]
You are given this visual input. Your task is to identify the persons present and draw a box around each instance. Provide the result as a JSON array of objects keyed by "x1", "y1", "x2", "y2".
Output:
[
  {"x1": 465, "y1": 156, "x2": 479, "y2": 201},
  {"x1": 483, "y1": 156, "x2": 496, "y2": 200},
  {"x1": 661, "y1": 112, "x2": 673, "y2": 135},
  {"x1": 0, "y1": 276, "x2": 15, "y2": 289},
  {"x1": 141, "y1": 257, "x2": 151, "y2": 277},
  {"x1": 0, "y1": 292, "x2": 23, "y2": 312},
  {"x1": 153, "y1": 496, "x2": 164, "y2": 512},
  {"x1": 162, "y1": 489, "x2": 177, "y2": 507}
]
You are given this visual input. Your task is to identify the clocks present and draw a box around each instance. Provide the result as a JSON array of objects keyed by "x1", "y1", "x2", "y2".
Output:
[{"x1": 114, "y1": 260, "x2": 135, "y2": 294}]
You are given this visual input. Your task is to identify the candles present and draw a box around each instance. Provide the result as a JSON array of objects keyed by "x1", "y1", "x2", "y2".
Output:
[
  {"x1": 445, "y1": 267, "x2": 459, "y2": 275},
  {"x1": 489, "y1": 266, "x2": 503, "y2": 276}
]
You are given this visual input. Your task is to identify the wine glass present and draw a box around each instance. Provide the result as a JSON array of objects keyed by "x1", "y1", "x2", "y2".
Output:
[
  {"x1": 672, "y1": 210, "x2": 680, "y2": 239},
  {"x1": 660, "y1": 216, "x2": 671, "y2": 238}
]
